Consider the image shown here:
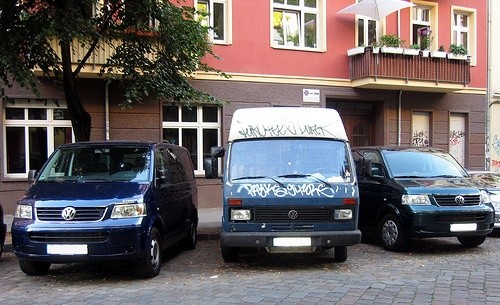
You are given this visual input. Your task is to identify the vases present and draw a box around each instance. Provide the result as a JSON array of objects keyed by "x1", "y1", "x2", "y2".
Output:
[
  {"x1": 347, "y1": 47, "x2": 365, "y2": 57},
  {"x1": 373, "y1": 46, "x2": 380, "y2": 54},
  {"x1": 381, "y1": 47, "x2": 468, "y2": 61}
]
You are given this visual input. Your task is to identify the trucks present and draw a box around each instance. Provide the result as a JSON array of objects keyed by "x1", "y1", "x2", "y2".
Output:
[{"x1": 205, "y1": 107, "x2": 373, "y2": 263}]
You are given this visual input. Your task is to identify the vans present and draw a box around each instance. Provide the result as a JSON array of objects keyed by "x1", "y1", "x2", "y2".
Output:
[
  {"x1": 11, "y1": 141, "x2": 198, "y2": 279},
  {"x1": 351, "y1": 145, "x2": 495, "y2": 252}
]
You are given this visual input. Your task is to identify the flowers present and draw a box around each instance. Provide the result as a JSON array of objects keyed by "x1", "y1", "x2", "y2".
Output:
[{"x1": 417, "y1": 30, "x2": 434, "y2": 50}]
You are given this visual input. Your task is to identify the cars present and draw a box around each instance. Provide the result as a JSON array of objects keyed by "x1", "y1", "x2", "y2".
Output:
[{"x1": 478, "y1": 188, "x2": 500, "y2": 228}]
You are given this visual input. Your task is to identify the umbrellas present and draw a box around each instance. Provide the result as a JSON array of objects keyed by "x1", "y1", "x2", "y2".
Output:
[
  {"x1": 305, "y1": 19, "x2": 315, "y2": 26},
  {"x1": 336, "y1": 0, "x2": 416, "y2": 21}
]
